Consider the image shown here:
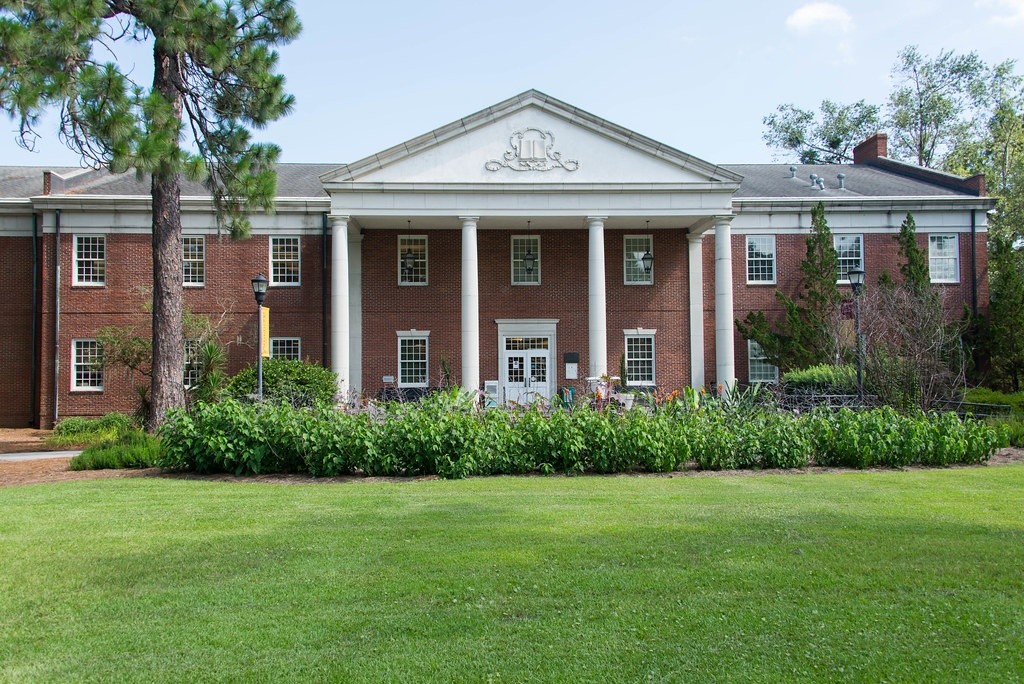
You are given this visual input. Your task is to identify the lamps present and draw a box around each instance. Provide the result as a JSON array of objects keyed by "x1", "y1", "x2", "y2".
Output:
[
  {"x1": 524, "y1": 220, "x2": 534, "y2": 274},
  {"x1": 404, "y1": 217, "x2": 414, "y2": 271},
  {"x1": 642, "y1": 221, "x2": 653, "y2": 274}
]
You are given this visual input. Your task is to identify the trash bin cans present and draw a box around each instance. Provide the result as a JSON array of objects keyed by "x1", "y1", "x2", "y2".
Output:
[{"x1": 561, "y1": 386, "x2": 575, "y2": 410}]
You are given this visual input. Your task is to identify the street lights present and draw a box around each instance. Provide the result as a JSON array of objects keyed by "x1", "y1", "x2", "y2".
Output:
[
  {"x1": 251, "y1": 272, "x2": 271, "y2": 412},
  {"x1": 847, "y1": 264, "x2": 868, "y2": 405}
]
